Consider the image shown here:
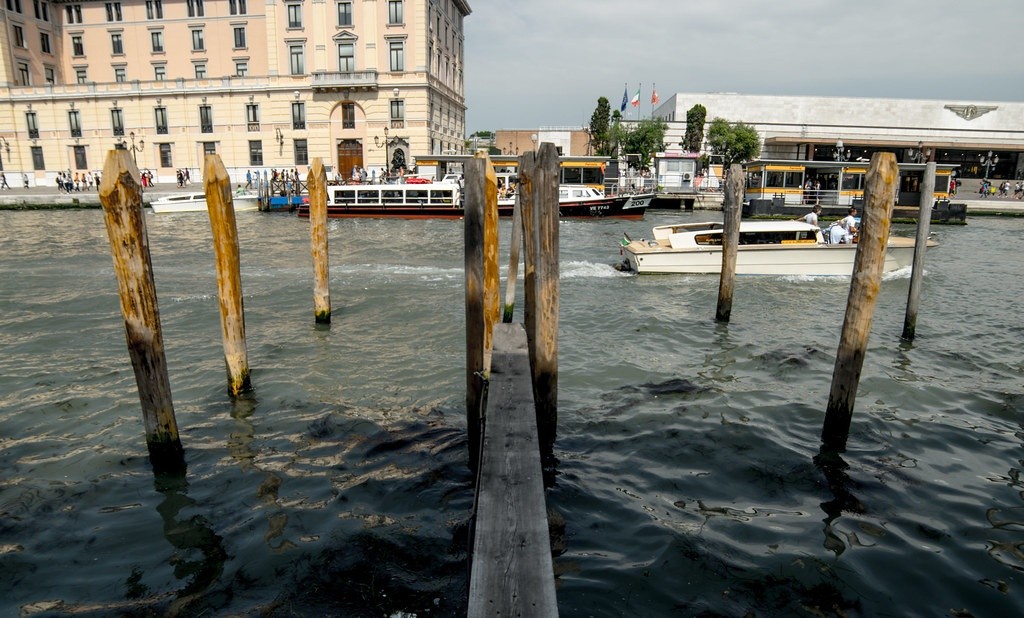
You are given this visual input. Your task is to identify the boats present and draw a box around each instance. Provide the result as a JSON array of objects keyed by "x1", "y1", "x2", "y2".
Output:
[
  {"x1": 149, "y1": 192, "x2": 258, "y2": 213},
  {"x1": 617, "y1": 220, "x2": 941, "y2": 276},
  {"x1": 411, "y1": 154, "x2": 611, "y2": 197},
  {"x1": 295, "y1": 171, "x2": 657, "y2": 220},
  {"x1": 258, "y1": 167, "x2": 303, "y2": 212},
  {"x1": 743, "y1": 159, "x2": 967, "y2": 226}
]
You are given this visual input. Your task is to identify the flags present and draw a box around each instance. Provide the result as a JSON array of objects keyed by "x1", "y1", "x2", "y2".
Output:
[
  {"x1": 621, "y1": 87, "x2": 628, "y2": 112},
  {"x1": 651, "y1": 85, "x2": 660, "y2": 105},
  {"x1": 631, "y1": 88, "x2": 640, "y2": 107}
]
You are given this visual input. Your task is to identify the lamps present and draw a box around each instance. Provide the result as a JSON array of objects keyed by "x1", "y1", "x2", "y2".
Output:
[{"x1": 275, "y1": 128, "x2": 283, "y2": 144}]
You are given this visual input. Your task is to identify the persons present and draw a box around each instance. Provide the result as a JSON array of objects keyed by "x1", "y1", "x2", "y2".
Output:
[
  {"x1": 0, "y1": 172, "x2": 11, "y2": 189},
  {"x1": 494, "y1": 167, "x2": 519, "y2": 201},
  {"x1": 24, "y1": 174, "x2": 29, "y2": 188},
  {"x1": 336, "y1": 165, "x2": 404, "y2": 184},
  {"x1": 448, "y1": 169, "x2": 454, "y2": 174},
  {"x1": 798, "y1": 205, "x2": 822, "y2": 240},
  {"x1": 949, "y1": 178, "x2": 1024, "y2": 201},
  {"x1": 139, "y1": 170, "x2": 154, "y2": 188},
  {"x1": 236, "y1": 169, "x2": 299, "y2": 205},
  {"x1": 837, "y1": 208, "x2": 859, "y2": 245},
  {"x1": 176, "y1": 168, "x2": 192, "y2": 187},
  {"x1": 57, "y1": 168, "x2": 102, "y2": 194},
  {"x1": 805, "y1": 175, "x2": 839, "y2": 205}
]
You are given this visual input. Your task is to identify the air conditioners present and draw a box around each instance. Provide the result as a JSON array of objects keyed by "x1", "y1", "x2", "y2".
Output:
[{"x1": 683, "y1": 173, "x2": 692, "y2": 181}]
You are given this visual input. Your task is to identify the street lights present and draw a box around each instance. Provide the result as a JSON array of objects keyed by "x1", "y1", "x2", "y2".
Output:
[
  {"x1": 907, "y1": 141, "x2": 932, "y2": 164},
  {"x1": 980, "y1": 150, "x2": 999, "y2": 179},
  {"x1": 531, "y1": 133, "x2": 537, "y2": 164},
  {"x1": 122, "y1": 132, "x2": 145, "y2": 166},
  {"x1": 832, "y1": 138, "x2": 851, "y2": 162},
  {"x1": 374, "y1": 126, "x2": 399, "y2": 181}
]
